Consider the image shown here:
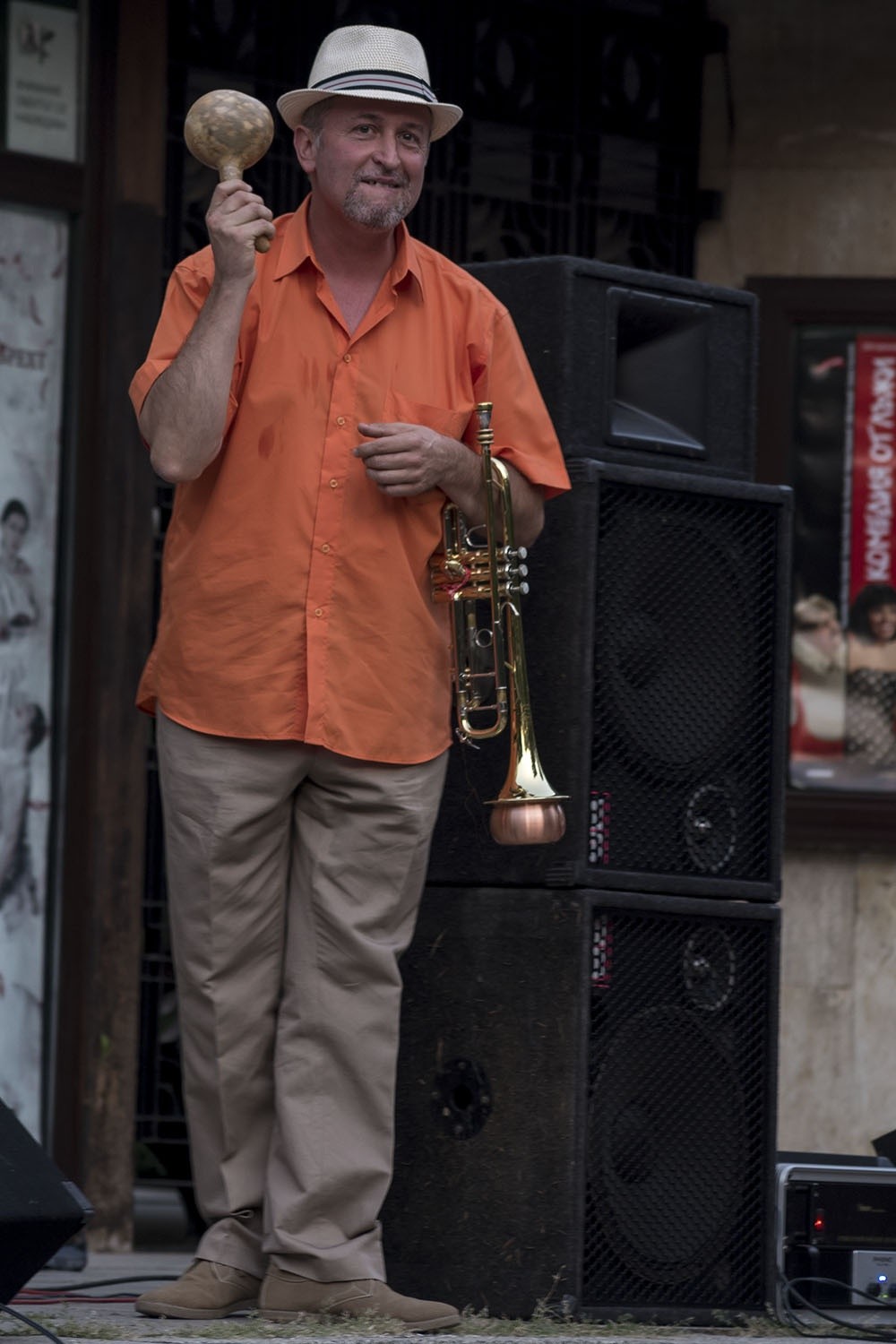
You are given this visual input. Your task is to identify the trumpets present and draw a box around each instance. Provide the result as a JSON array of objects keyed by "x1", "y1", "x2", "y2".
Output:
[{"x1": 425, "y1": 401, "x2": 573, "y2": 847}]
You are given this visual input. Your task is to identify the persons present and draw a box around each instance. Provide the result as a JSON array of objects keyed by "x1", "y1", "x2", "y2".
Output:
[
  {"x1": 789, "y1": 586, "x2": 896, "y2": 765},
  {"x1": 0, "y1": 499, "x2": 47, "y2": 916},
  {"x1": 128, "y1": 25, "x2": 572, "y2": 1331}
]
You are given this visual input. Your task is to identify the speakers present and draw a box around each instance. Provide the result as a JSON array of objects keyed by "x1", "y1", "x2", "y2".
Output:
[{"x1": 381, "y1": 256, "x2": 800, "y2": 1327}]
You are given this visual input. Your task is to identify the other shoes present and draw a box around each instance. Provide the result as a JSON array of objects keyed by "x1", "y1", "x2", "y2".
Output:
[
  {"x1": 260, "y1": 1261, "x2": 461, "y2": 1330},
  {"x1": 136, "y1": 1259, "x2": 259, "y2": 1318}
]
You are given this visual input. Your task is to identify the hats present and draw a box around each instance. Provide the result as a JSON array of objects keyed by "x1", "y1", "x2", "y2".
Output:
[{"x1": 277, "y1": 25, "x2": 463, "y2": 142}]
